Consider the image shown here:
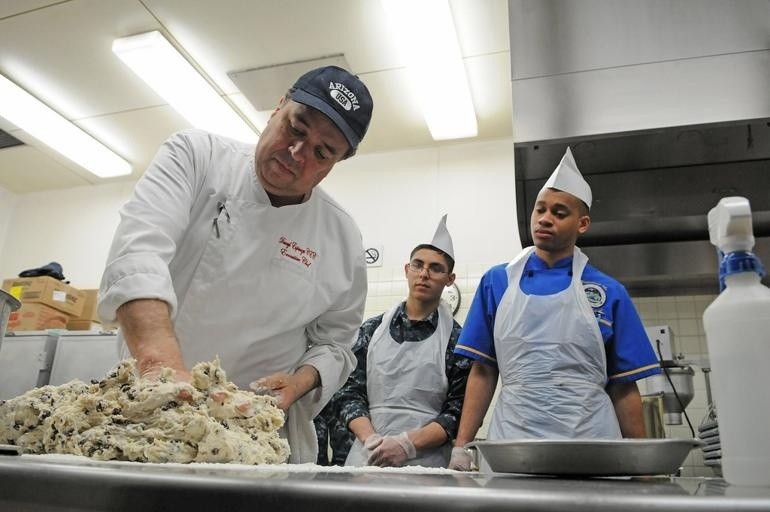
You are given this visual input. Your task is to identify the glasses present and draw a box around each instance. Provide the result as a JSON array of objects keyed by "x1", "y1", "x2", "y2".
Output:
[{"x1": 409, "y1": 261, "x2": 450, "y2": 277}]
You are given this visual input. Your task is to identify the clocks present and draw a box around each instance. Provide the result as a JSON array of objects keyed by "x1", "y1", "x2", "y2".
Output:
[{"x1": 437, "y1": 278, "x2": 462, "y2": 319}]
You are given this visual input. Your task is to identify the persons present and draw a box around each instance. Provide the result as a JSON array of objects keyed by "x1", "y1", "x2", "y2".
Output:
[
  {"x1": 447, "y1": 188, "x2": 664, "y2": 473},
  {"x1": 97, "y1": 66, "x2": 367, "y2": 465},
  {"x1": 331, "y1": 245, "x2": 482, "y2": 467}
]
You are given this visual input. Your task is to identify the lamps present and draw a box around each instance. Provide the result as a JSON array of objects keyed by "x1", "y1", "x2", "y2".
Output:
[
  {"x1": 112, "y1": 25, "x2": 265, "y2": 149},
  {"x1": 0, "y1": 67, "x2": 134, "y2": 182}
]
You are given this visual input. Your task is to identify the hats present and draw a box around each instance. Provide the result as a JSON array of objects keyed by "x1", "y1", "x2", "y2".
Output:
[
  {"x1": 543, "y1": 147, "x2": 592, "y2": 212},
  {"x1": 410, "y1": 214, "x2": 455, "y2": 263},
  {"x1": 294, "y1": 65, "x2": 374, "y2": 149}
]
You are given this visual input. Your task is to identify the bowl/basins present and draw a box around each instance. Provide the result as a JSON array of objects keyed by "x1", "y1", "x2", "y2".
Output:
[
  {"x1": 697, "y1": 420, "x2": 722, "y2": 477},
  {"x1": 465, "y1": 436, "x2": 707, "y2": 476}
]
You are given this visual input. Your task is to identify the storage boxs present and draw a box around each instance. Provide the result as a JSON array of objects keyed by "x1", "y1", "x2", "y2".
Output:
[{"x1": 1, "y1": 274, "x2": 104, "y2": 334}]
